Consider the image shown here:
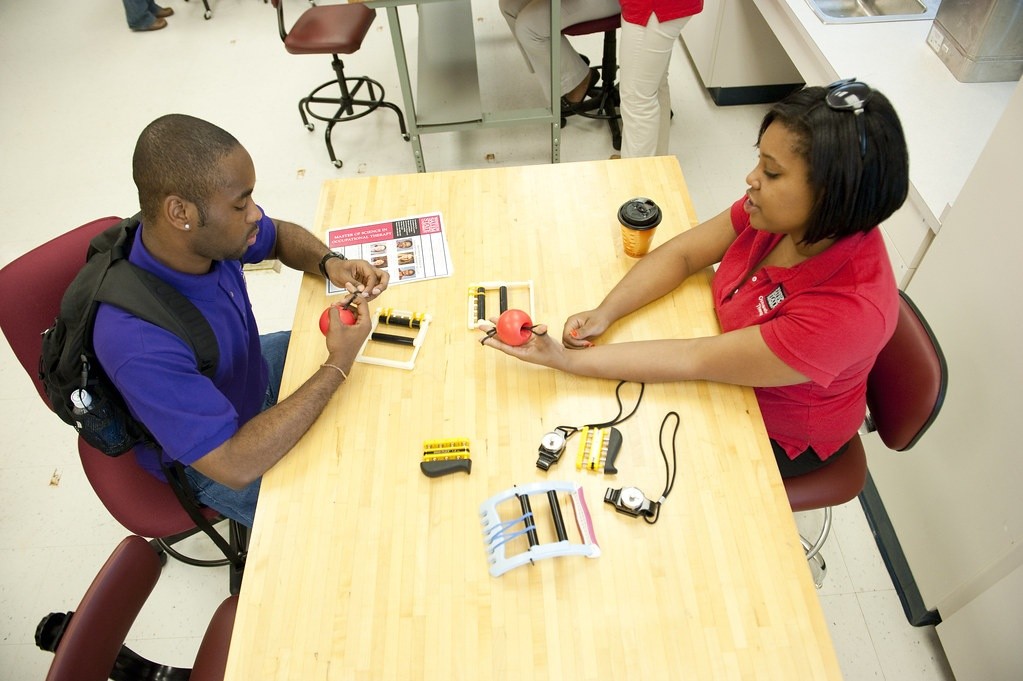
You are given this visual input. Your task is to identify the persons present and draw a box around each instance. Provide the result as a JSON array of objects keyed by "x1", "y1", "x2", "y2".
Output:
[
  {"x1": 397, "y1": 240, "x2": 412, "y2": 248},
  {"x1": 92, "y1": 115, "x2": 390, "y2": 527},
  {"x1": 371, "y1": 244, "x2": 386, "y2": 254},
  {"x1": 371, "y1": 257, "x2": 386, "y2": 267},
  {"x1": 610, "y1": 0, "x2": 705, "y2": 159},
  {"x1": 473, "y1": 77, "x2": 909, "y2": 479},
  {"x1": 398, "y1": 253, "x2": 414, "y2": 265},
  {"x1": 122, "y1": 0, "x2": 174, "y2": 32},
  {"x1": 399, "y1": 268, "x2": 415, "y2": 280},
  {"x1": 498, "y1": 0, "x2": 621, "y2": 111}
]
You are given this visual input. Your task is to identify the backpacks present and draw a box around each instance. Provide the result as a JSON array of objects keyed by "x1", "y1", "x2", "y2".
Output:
[{"x1": 37, "y1": 211, "x2": 219, "y2": 458}]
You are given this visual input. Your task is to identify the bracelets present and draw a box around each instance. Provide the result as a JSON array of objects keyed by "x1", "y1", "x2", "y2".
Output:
[{"x1": 320, "y1": 363, "x2": 347, "y2": 380}]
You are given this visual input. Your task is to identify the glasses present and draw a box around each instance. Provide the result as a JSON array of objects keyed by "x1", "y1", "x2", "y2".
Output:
[{"x1": 824, "y1": 78, "x2": 868, "y2": 160}]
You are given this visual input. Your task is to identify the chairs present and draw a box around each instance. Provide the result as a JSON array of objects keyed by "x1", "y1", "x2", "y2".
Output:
[
  {"x1": 0, "y1": 218, "x2": 260, "y2": 593},
  {"x1": 36, "y1": 535, "x2": 239, "y2": 681},
  {"x1": 780, "y1": 283, "x2": 950, "y2": 569}
]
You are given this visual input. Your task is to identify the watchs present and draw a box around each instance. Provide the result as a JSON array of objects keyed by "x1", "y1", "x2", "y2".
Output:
[{"x1": 318, "y1": 251, "x2": 347, "y2": 279}]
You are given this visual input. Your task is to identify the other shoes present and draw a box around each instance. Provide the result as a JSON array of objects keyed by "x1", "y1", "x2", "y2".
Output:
[
  {"x1": 133, "y1": 18, "x2": 167, "y2": 31},
  {"x1": 561, "y1": 68, "x2": 600, "y2": 110},
  {"x1": 154, "y1": 8, "x2": 173, "y2": 17},
  {"x1": 579, "y1": 53, "x2": 591, "y2": 66}
]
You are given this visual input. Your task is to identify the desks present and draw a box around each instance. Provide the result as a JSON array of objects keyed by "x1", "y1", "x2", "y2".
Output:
[
  {"x1": 348, "y1": 0, "x2": 563, "y2": 172},
  {"x1": 220, "y1": 156, "x2": 842, "y2": 681}
]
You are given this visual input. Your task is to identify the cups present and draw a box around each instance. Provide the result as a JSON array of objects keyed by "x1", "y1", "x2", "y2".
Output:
[{"x1": 617, "y1": 197, "x2": 663, "y2": 258}]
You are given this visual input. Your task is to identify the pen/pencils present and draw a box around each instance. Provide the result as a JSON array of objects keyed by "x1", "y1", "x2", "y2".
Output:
[
  {"x1": 379, "y1": 315, "x2": 420, "y2": 329},
  {"x1": 500, "y1": 287, "x2": 507, "y2": 314},
  {"x1": 478, "y1": 287, "x2": 485, "y2": 320},
  {"x1": 371, "y1": 333, "x2": 416, "y2": 347},
  {"x1": 547, "y1": 490, "x2": 568, "y2": 541},
  {"x1": 518, "y1": 494, "x2": 540, "y2": 548}
]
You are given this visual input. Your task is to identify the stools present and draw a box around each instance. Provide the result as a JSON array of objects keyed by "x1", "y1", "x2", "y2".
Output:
[
  {"x1": 561, "y1": 13, "x2": 672, "y2": 148},
  {"x1": 269, "y1": 0, "x2": 410, "y2": 170}
]
